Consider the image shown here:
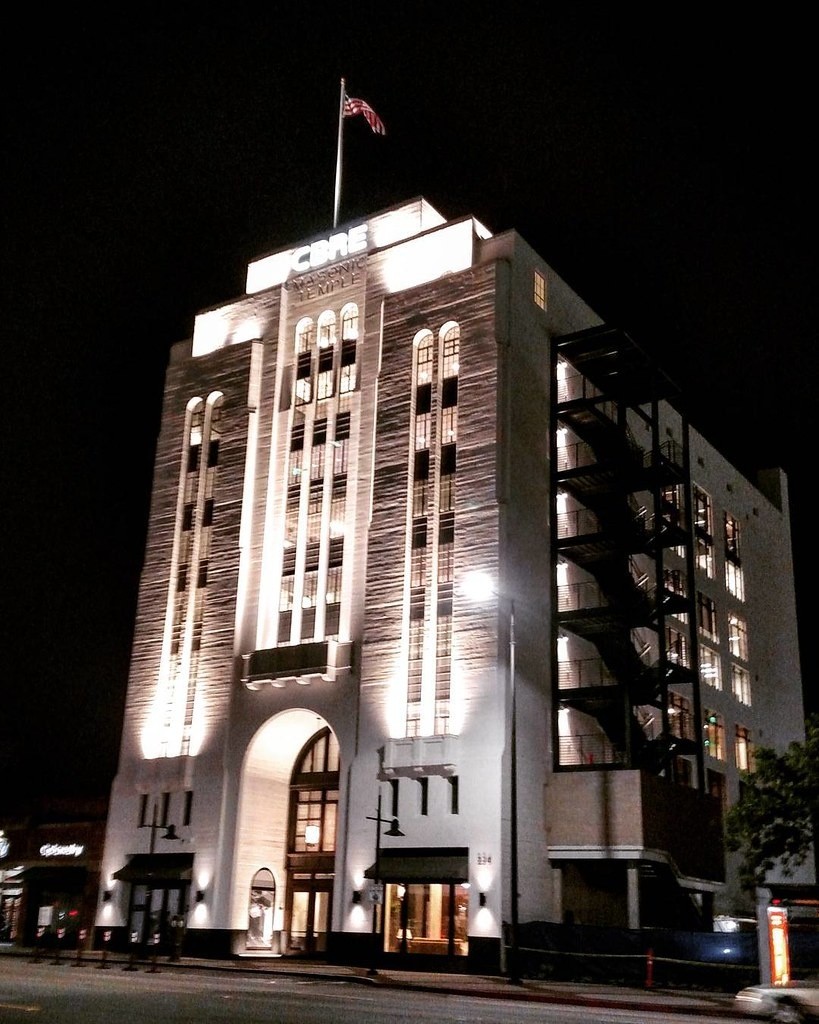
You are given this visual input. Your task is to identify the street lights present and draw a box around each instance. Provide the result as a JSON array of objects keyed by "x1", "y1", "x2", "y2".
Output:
[
  {"x1": 139, "y1": 805, "x2": 180, "y2": 959},
  {"x1": 366, "y1": 786, "x2": 406, "y2": 976}
]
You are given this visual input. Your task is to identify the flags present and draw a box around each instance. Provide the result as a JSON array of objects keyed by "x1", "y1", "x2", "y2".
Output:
[{"x1": 344, "y1": 91, "x2": 385, "y2": 135}]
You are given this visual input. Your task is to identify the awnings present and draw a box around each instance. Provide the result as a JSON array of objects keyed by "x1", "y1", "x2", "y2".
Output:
[
  {"x1": 365, "y1": 855, "x2": 468, "y2": 884},
  {"x1": 114, "y1": 855, "x2": 194, "y2": 888},
  {"x1": 0, "y1": 866, "x2": 86, "y2": 890}
]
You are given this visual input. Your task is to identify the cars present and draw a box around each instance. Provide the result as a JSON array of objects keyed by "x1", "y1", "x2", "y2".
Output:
[{"x1": 733, "y1": 980, "x2": 819, "y2": 1024}]
